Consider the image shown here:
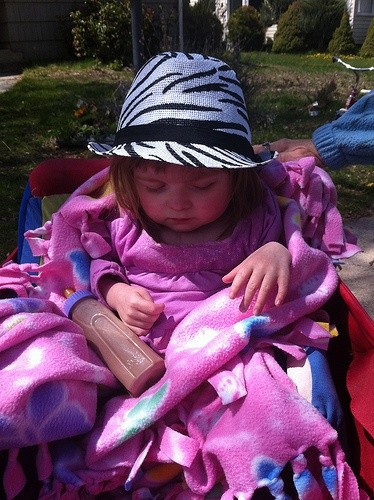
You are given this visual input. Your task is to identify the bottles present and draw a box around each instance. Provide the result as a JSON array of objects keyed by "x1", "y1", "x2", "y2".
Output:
[{"x1": 62, "y1": 287, "x2": 166, "y2": 397}]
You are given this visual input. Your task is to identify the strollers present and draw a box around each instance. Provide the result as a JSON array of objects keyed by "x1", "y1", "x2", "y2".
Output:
[{"x1": 3, "y1": 156, "x2": 374, "y2": 500}]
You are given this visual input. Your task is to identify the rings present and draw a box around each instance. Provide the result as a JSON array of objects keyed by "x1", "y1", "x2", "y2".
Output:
[{"x1": 262, "y1": 141, "x2": 272, "y2": 152}]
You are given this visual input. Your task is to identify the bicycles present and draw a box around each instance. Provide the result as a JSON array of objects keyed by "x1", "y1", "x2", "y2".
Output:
[{"x1": 330, "y1": 55, "x2": 374, "y2": 123}]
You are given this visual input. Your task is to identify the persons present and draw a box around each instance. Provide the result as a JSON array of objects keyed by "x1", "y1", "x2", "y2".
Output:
[
  {"x1": 84, "y1": 50, "x2": 293, "y2": 361},
  {"x1": 252, "y1": 89, "x2": 373, "y2": 173}
]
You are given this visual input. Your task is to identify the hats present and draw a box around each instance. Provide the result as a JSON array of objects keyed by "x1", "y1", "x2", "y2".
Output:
[{"x1": 89, "y1": 51, "x2": 278, "y2": 170}]
